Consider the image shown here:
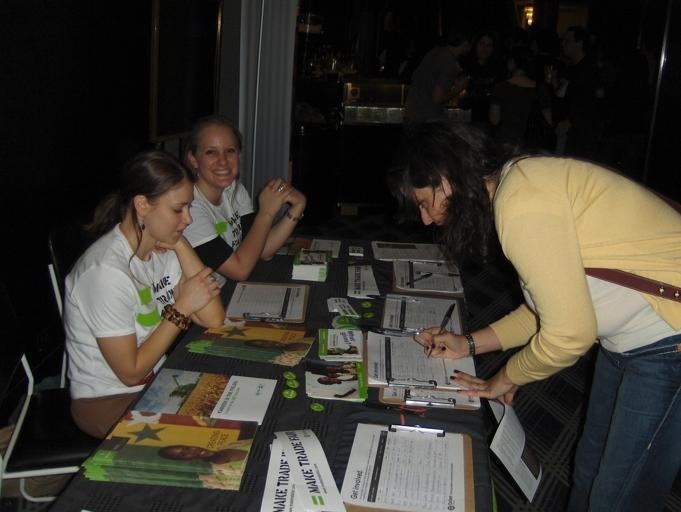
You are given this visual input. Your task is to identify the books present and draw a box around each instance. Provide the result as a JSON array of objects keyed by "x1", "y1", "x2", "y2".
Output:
[{"x1": 487, "y1": 398, "x2": 543, "y2": 503}]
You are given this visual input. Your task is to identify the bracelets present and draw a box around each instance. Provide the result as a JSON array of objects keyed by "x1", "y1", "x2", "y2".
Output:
[{"x1": 285, "y1": 209, "x2": 305, "y2": 224}]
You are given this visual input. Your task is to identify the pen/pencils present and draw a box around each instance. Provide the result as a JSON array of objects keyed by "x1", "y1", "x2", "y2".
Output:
[
  {"x1": 406, "y1": 273, "x2": 432, "y2": 286},
  {"x1": 367, "y1": 295, "x2": 422, "y2": 302},
  {"x1": 427, "y1": 304, "x2": 456, "y2": 358},
  {"x1": 416, "y1": 271, "x2": 460, "y2": 277},
  {"x1": 363, "y1": 402, "x2": 413, "y2": 414}
]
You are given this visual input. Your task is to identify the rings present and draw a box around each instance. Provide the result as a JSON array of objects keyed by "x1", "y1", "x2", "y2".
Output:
[{"x1": 278, "y1": 184, "x2": 286, "y2": 193}]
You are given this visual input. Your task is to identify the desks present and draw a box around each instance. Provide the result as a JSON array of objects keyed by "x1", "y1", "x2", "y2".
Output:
[{"x1": 46, "y1": 235, "x2": 498, "y2": 512}]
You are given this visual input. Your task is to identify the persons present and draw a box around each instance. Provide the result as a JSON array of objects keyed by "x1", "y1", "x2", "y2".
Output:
[
  {"x1": 390, "y1": 114, "x2": 681, "y2": 511},
  {"x1": 63, "y1": 149, "x2": 229, "y2": 440},
  {"x1": 172, "y1": 113, "x2": 308, "y2": 283}
]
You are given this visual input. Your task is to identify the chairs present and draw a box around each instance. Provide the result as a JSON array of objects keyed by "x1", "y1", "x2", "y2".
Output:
[
  {"x1": 47, "y1": 222, "x2": 88, "y2": 388},
  {"x1": 1, "y1": 281, "x2": 105, "y2": 503}
]
